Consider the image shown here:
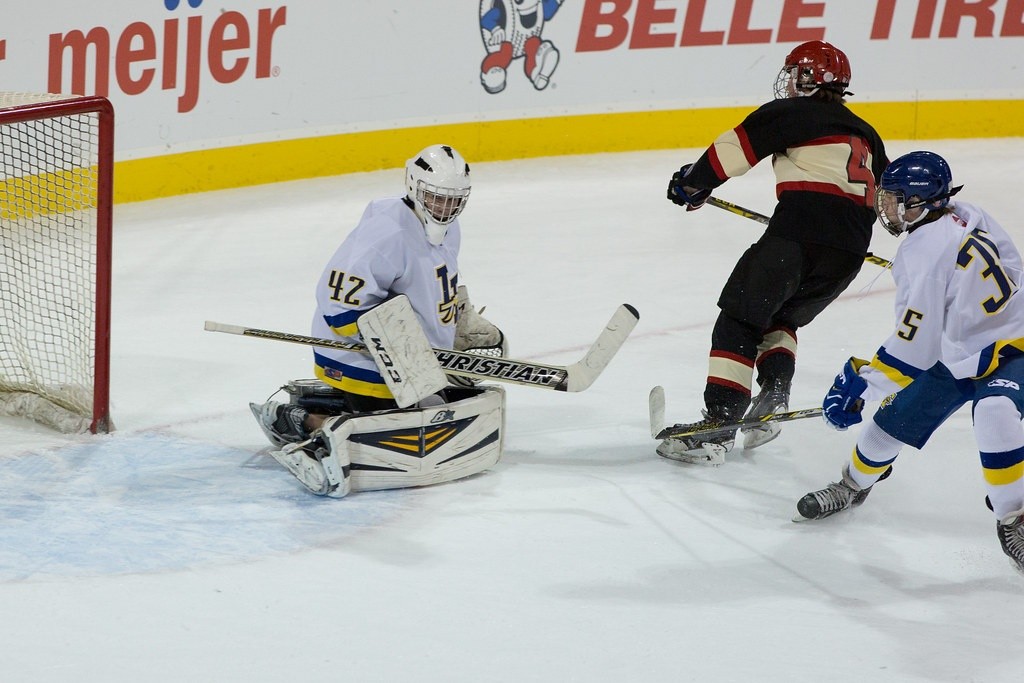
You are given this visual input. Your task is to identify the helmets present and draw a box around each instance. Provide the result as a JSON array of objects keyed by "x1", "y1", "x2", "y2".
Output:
[
  {"x1": 873, "y1": 151, "x2": 952, "y2": 238},
  {"x1": 773, "y1": 40, "x2": 851, "y2": 99},
  {"x1": 403, "y1": 143, "x2": 472, "y2": 246}
]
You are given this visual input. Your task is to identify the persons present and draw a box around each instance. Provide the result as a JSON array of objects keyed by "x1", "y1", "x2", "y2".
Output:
[
  {"x1": 655, "y1": 40, "x2": 889, "y2": 467},
  {"x1": 791, "y1": 151, "x2": 1024, "y2": 569},
  {"x1": 248, "y1": 145, "x2": 510, "y2": 496}
]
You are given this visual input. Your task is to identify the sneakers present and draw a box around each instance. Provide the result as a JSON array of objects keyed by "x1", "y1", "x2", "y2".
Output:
[
  {"x1": 741, "y1": 375, "x2": 792, "y2": 448},
  {"x1": 985, "y1": 495, "x2": 1024, "y2": 572},
  {"x1": 249, "y1": 400, "x2": 310, "y2": 446},
  {"x1": 270, "y1": 433, "x2": 328, "y2": 491},
  {"x1": 655, "y1": 415, "x2": 740, "y2": 465},
  {"x1": 792, "y1": 461, "x2": 892, "y2": 523}
]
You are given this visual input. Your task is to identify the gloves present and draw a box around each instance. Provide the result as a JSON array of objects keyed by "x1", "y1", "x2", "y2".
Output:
[
  {"x1": 823, "y1": 357, "x2": 871, "y2": 432},
  {"x1": 666, "y1": 163, "x2": 713, "y2": 211}
]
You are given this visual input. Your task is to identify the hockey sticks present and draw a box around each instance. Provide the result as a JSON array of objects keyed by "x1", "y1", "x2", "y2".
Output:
[
  {"x1": 706, "y1": 195, "x2": 894, "y2": 272},
  {"x1": 198, "y1": 299, "x2": 644, "y2": 397},
  {"x1": 647, "y1": 383, "x2": 866, "y2": 442}
]
